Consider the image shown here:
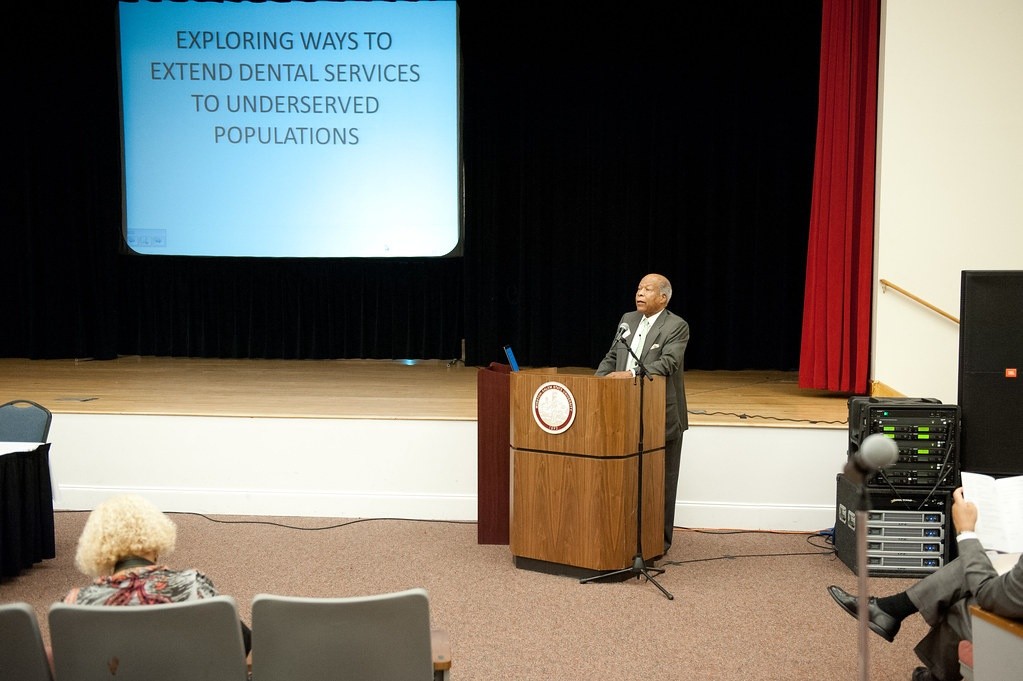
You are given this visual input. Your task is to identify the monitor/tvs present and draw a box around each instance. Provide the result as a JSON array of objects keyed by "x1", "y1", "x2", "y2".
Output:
[{"x1": 503, "y1": 345, "x2": 519, "y2": 372}]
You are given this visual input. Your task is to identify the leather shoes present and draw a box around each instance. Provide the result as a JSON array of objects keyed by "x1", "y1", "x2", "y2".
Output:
[
  {"x1": 827, "y1": 586, "x2": 901, "y2": 644},
  {"x1": 911, "y1": 666, "x2": 938, "y2": 680}
]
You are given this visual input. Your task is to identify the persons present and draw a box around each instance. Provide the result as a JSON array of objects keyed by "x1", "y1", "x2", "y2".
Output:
[
  {"x1": 59, "y1": 494, "x2": 252, "y2": 659},
  {"x1": 826, "y1": 486, "x2": 1023, "y2": 680},
  {"x1": 592, "y1": 273, "x2": 689, "y2": 558}
]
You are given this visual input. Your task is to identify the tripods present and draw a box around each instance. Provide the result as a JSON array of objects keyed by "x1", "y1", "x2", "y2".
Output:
[{"x1": 581, "y1": 333, "x2": 673, "y2": 600}]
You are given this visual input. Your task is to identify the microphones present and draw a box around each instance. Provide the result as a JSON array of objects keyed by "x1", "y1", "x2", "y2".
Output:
[
  {"x1": 844, "y1": 434, "x2": 898, "y2": 485},
  {"x1": 611, "y1": 322, "x2": 629, "y2": 349}
]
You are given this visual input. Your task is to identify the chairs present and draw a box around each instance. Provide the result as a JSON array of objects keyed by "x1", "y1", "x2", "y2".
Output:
[
  {"x1": 48, "y1": 595, "x2": 252, "y2": 681},
  {"x1": 0, "y1": 399, "x2": 52, "y2": 444},
  {"x1": 959, "y1": 605, "x2": 1023, "y2": 681},
  {"x1": 0, "y1": 602, "x2": 55, "y2": 681},
  {"x1": 251, "y1": 589, "x2": 452, "y2": 681}
]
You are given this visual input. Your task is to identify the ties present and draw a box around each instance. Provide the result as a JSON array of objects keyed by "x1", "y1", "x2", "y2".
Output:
[{"x1": 628, "y1": 319, "x2": 647, "y2": 368}]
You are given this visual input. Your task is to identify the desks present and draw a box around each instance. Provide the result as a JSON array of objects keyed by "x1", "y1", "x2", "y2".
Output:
[
  {"x1": 509, "y1": 366, "x2": 667, "y2": 582},
  {"x1": 477, "y1": 361, "x2": 522, "y2": 544},
  {"x1": 0, "y1": 441, "x2": 57, "y2": 584}
]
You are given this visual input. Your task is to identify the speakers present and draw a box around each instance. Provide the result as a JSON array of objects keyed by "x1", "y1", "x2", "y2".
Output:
[{"x1": 957, "y1": 271, "x2": 1023, "y2": 479}]
